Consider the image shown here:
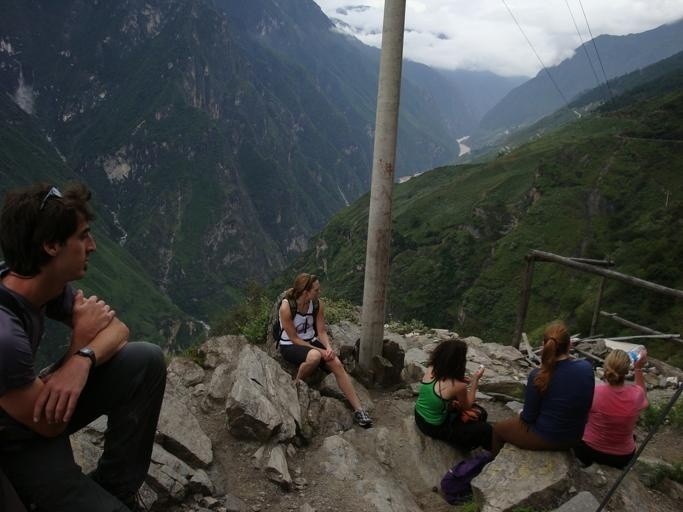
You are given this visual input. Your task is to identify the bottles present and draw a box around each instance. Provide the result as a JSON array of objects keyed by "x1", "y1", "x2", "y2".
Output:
[{"x1": 626, "y1": 344, "x2": 646, "y2": 364}]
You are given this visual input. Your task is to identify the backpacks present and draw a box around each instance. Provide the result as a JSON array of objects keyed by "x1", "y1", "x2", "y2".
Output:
[{"x1": 272, "y1": 296, "x2": 320, "y2": 349}]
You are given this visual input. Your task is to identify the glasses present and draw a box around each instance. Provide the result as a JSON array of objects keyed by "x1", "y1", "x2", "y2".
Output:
[{"x1": 40, "y1": 186, "x2": 65, "y2": 208}]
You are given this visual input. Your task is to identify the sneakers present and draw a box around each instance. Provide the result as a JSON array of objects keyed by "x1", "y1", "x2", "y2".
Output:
[
  {"x1": 354, "y1": 409, "x2": 373, "y2": 429},
  {"x1": 86, "y1": 469, "x2": 144, "y2": 512}
]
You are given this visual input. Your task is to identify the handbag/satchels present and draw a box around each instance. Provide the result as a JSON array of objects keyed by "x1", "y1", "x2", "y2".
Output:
[
  {"x1": 440, "y1": 451, "x2": 493, "y2": 506},
  {"x1": 448, "y1": 399, "x2": 488, "y2": 429}
]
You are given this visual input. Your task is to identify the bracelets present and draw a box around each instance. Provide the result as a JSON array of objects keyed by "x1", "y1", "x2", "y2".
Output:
[{"x1": 634, "y1": 369, "x2": 642, "y2": 374}]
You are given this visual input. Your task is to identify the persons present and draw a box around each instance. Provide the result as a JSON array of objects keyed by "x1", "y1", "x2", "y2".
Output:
[
  {"x1": 493, "y1": 325, "x2": 595, "y2": 450},
  {"x1": 0, "y1": 186, "x2": 167, "y2": 511},
  {"x1": 273, "y1": 273, "x2": 373, "y2": 427},
  {"x1": 577, "y1": 349, "x2": 651, "y2": 470},
  {"x1": 415, "y1": 340, "x2": 488, "y2": 440}
]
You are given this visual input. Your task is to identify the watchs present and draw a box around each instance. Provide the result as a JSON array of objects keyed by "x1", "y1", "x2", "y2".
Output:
[{"x1": 73, "y1": 347, "x2": 98, "y2": 367}]
